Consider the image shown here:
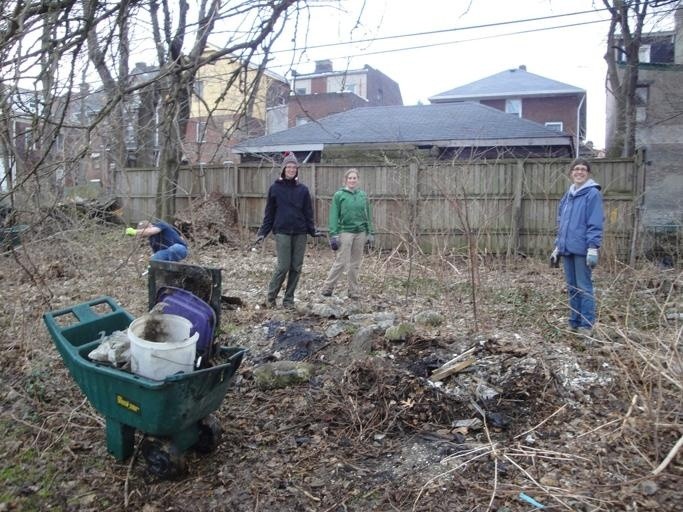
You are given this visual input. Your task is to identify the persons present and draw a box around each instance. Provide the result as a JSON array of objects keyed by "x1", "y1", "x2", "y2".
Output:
[
  {"x1": 255, "y1": 152, "x2": 327, "y2": 309},
  {"x1": 321, "y1": 169, "x2": 375, "y2": 298},
  {"x1": 125, "y1": 220, "x2": 187, "y2": 261},
  {"x1": 550, "y1": 159, "x2": 603, "y2": 336}
]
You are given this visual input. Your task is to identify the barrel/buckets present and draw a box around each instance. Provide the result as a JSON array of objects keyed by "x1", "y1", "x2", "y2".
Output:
[{"x1": 128, "y1": 312, "x2": 203, "y2": 381}]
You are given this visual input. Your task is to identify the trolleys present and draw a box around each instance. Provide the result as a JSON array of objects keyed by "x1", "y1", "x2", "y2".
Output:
[{"x1": 43, "y1": 296, "x2": 250, "y2": 483}]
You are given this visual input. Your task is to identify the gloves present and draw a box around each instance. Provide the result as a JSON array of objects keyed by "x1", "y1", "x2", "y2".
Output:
[
  {"x1": 329, "y1": 237, "x2": 339, "y2": 250},
  {"x1": 255, "y1": 235, "x2": 264, "y2": 245},
  {"x1": 551, "y1": 247, "x2": 559, "y2": 263},
  {"x1": 366, "y1": 234, "x2": 375, "y2": 249},
  {"x1": 586, "y1": 248, "x2": 599, "y2": 268},
  {"x1": 126, "y1": 227, "x2": 136, "y2": 236},
  {"x1": 313, "y1": 232, "x2": 327, "y2": 238}
]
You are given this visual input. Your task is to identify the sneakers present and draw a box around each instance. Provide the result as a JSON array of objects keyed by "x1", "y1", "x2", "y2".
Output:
[
  {"x1": 284, "y1": 304, "x2": 296, "y2": 309},
  {"x1": 579, "y1": 328, "x2": 594, "y2": 339},
  {"x1": 322, "y1": 290, "x2": 332, "y2": 296},
  {"x1": 266, "y1": 300, "x2": 276, "y2": 309},
  {"x1": 349, "y1": 291, "x2": 360, "y2": 297}
]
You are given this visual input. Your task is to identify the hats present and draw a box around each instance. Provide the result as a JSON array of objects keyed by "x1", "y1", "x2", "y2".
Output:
[{"x1": 278, "y1": 152, "x2": 299, "y2": 186}]
